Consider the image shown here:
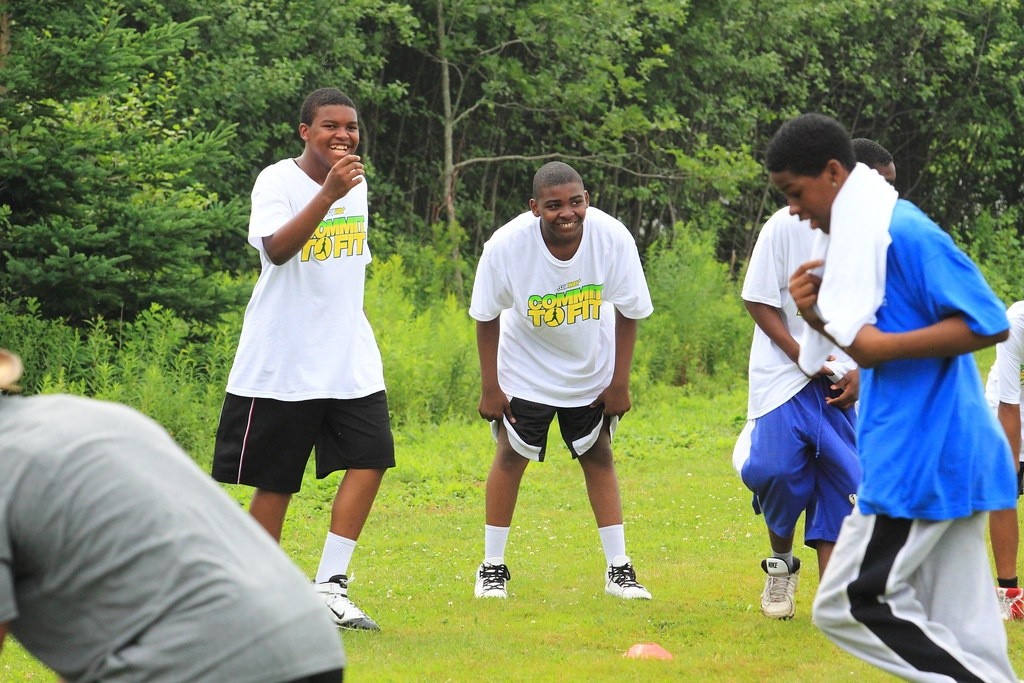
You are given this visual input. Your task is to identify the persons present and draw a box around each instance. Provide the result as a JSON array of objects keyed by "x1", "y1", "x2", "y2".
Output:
[
  {"x1": 732, "y1": 138, "x2": 895, "y2": 620},
  {"x1": 984, "y1": 300, "x2": 1024, "y2": 621},
  {"x1": 467, "y1": 162, "x2": 653, "y2": 598},
  {"x1": 211, "y1": 87, "x2": 397, "y2": 630},
  {"x1": 1, "y1": 346, "x2": 348, "y2": 683},
  {"x1": 766, "y1": 111, "x2": 1018, "y2": 683}
]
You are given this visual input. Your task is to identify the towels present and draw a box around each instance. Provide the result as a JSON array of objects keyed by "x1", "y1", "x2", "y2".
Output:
[{"x1": 796, "y1": 160, "x2": 899, "y2": 380}]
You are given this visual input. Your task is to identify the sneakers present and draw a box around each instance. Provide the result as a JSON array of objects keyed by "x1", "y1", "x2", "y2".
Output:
[
  {"x1": 474, "y1": 556, "x2": 507, "y2": 600},
  {"x1": 605, "y1": 553, "x2": 653, "y2": 602},
  {"x1": 997, "y1": 588, "x2": 1024, "y2": 621},
  {"x1": 313, "y1": 573, "x2": 382, "y2": 636},
  {"x1": 759, "y1": 555, "x2": 801, "y2": 622}
]
